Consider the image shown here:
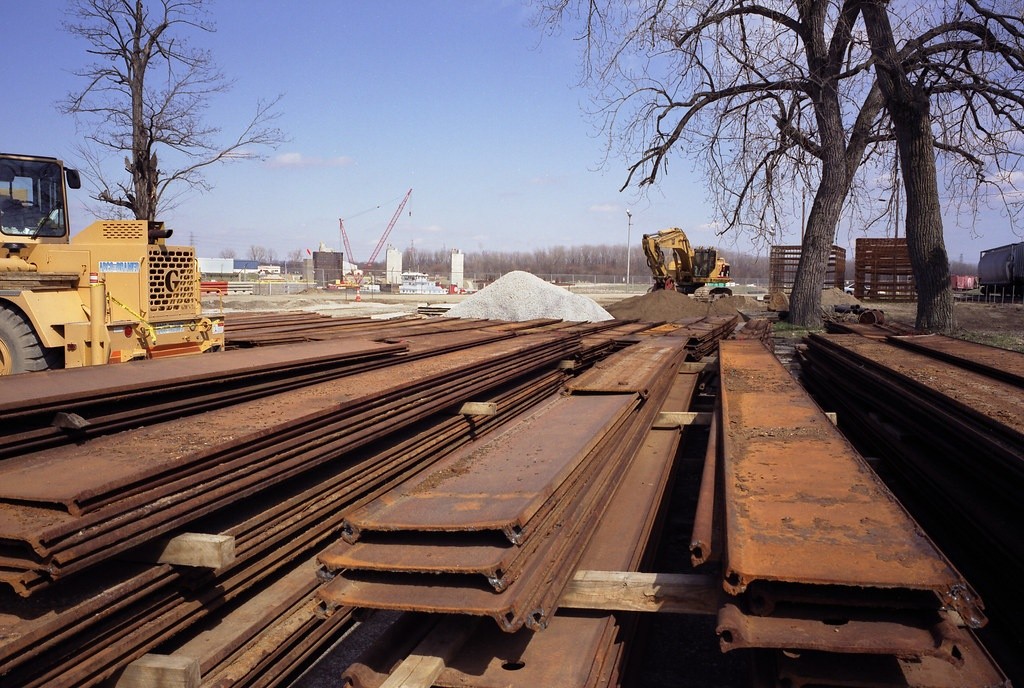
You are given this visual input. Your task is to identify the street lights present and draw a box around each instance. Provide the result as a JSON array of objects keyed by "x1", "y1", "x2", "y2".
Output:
[{"x1": 626, "y1": 208, "x2": 633, "y2": 285}]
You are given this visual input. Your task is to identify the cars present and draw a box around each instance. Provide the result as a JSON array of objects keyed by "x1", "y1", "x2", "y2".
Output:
[{"x1": 844, "y1": 282, "x2": 886, "y2": 301}]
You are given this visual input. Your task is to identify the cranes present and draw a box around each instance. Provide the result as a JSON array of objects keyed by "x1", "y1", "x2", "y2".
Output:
[{"x1": 338, "y1": 188, "x2": 414, "y2": 287}]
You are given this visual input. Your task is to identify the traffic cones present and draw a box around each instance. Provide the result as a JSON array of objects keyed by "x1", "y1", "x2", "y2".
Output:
[{"x1": 354, "y1": 287, "x2": 362, "y2": 302}]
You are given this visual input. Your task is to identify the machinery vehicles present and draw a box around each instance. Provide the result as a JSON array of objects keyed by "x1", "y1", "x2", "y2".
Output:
[
  {"x1": 0, "y1": 153, "x2": 228, "y2": 389},
  {"x1": 642, "y1": 227, "x2": 734, "y2": 303}
]
